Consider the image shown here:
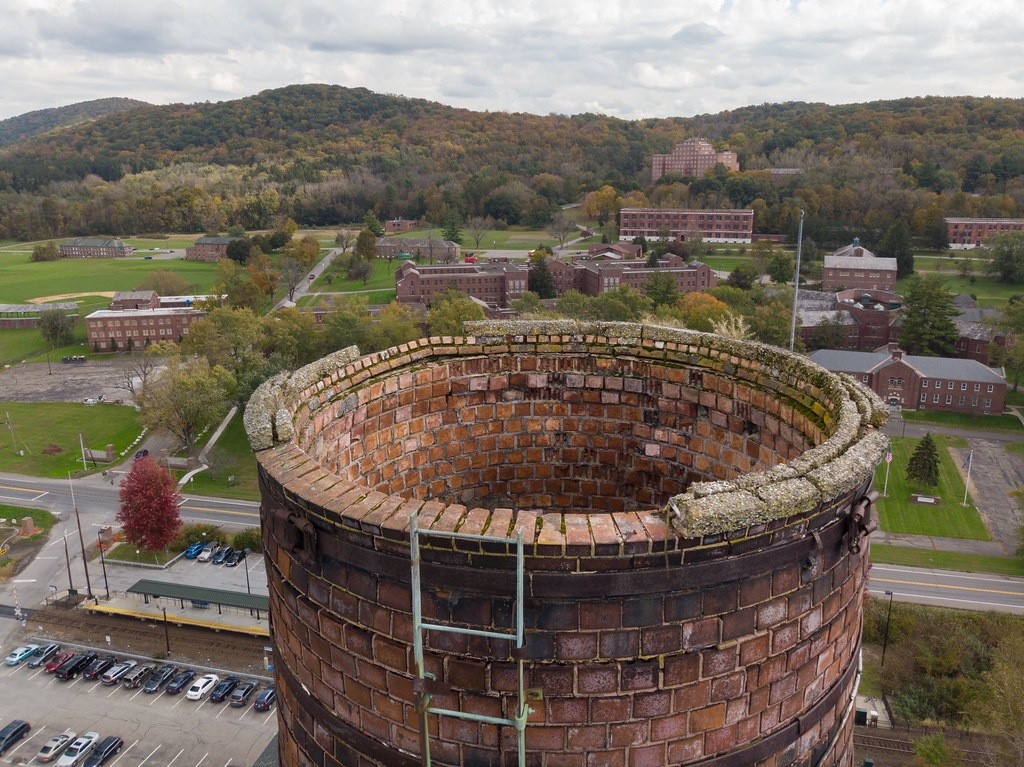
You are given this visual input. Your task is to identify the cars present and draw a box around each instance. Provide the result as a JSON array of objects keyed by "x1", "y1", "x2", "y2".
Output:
[
  {"x1": 0, "y1": 719, "x2": 30, "y2": 757},
  {"x1": 5, "y1": 643, "x2": 276, "y2": 712},
  {"x1": 134, "y1": 449, "x2": 148, "y2": 461},
  {"x1": 36, "y1": 731, "x2": 123, "y2": 767},
  {"x1": 186, "y1": 541, "x2": 246, "y2": 567}
]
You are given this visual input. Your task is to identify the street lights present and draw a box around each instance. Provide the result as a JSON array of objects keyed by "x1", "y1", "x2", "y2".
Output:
[
  {"x1": 156, "y1": 605, "x2": 171, "y2": 656},
  {"x1": 237, "y1": 548, "x2": 251, "y2": 594},
  {"x1": 98, "y1": 530, "x2": 109, "y2": 597},
  {"x1": 881, "y1": 590, "x2": 893, "y2": 667}
]
